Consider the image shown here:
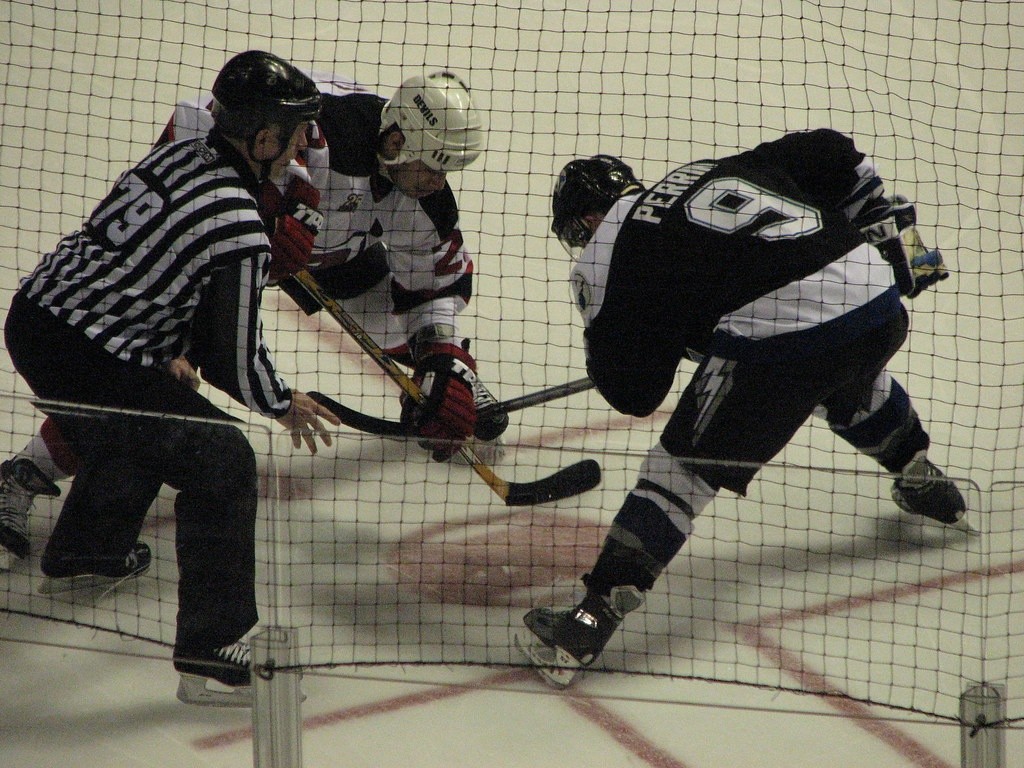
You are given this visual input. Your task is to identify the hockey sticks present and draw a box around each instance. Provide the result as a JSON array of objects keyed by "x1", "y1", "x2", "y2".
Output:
[
  {"x1": 288, "y1": 268, "x2": 603, "y2": 509},
  {"x1": 300, "y1": 374, "x2": 596, "y2": 441}
]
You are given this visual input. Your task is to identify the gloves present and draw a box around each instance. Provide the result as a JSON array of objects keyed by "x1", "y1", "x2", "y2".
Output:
[
  {"x1": 402, "y1": 341, "x2": 478, "y2": 463},
  {"x1": 863, "y1": 195, "x2": 947, "y2": 299},
  {"x1": 269, "y1": 178, "x2": 323, "y2": 277}
]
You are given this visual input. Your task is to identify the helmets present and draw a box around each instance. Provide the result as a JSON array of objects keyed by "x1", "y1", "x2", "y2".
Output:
[
  {"x1": 208, "y1": 50, "x2": 324, "y2": 164},
  {"x1": 551, "y1": 152, "x2": 646, "y2": 235},
  {"x1": 377, "y1": 70, "x2": 481, "y2": 178}
]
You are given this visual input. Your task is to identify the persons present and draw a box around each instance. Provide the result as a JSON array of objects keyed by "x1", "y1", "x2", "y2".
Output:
[
  {"x1": 1, "y1": 70, "x2": 508, "y2": 572},
  {"x1": 512, "y1": 128, "x2": 979, "y2": 688},
  {"x1": 4, "y1": 49, "x2": 342, "y2": 709}
]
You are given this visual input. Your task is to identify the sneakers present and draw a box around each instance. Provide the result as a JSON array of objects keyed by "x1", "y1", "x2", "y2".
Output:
[
  {"x1": 511, "y1": 594, "x2": 621, "y2": 689},
  {"x1": 171, "y1": 639, "x2": 307, "y2": 710},
  {"x1": 0, "y1": 455, "x2": 61, "y2": 570},
  {"x1": 32, "y1": 539, "x2": 154, "y2": 597},
  {"x1": 470, "y1": 374, "x2": 508, "y2": 440},
  {"x1": 889, "y1": 459, "x2": 974, "y2": 536}
]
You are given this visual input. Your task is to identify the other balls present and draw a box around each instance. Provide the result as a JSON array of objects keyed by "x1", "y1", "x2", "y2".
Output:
[{"x1": 433, "y1": 445, "x2": 459, "y2": 464}]
